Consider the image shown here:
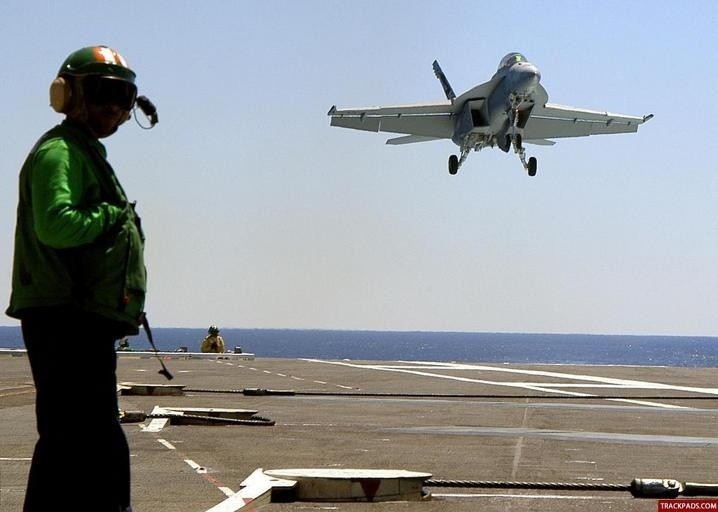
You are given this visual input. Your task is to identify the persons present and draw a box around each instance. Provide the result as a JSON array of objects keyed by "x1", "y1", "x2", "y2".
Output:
[
  {"x1": 6, "y1": 45, "x2": 148, "y2": 511},
  {"x1": 200, "y1": 326, "x2": 224, "y2": 353},
  {"x1": 121, "y1": 338, "x2": 129, "y2": 348}
]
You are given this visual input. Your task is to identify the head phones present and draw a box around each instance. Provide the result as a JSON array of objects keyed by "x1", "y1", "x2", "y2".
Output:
[{"x1": 49, "y1": 75, "x2": 133, "y2": 124}]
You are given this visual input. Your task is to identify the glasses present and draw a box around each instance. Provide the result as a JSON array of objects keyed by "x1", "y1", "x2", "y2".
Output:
[{"x1": 81, "y1": 77, "x2": 136, "y2": 111}]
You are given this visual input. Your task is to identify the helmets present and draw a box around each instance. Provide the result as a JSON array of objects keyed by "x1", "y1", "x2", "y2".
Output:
[
  {"x1": 58, "y1": 44, "x2": 138, "y2": 89},
  {"x1": 208, "y1": 326, "x2": 219, "y2": 334}
]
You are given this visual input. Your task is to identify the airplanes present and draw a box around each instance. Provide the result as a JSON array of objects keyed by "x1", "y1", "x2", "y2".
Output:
[{"x1": 322, "y1": 49, "x2": 656, "y2": 180}]
